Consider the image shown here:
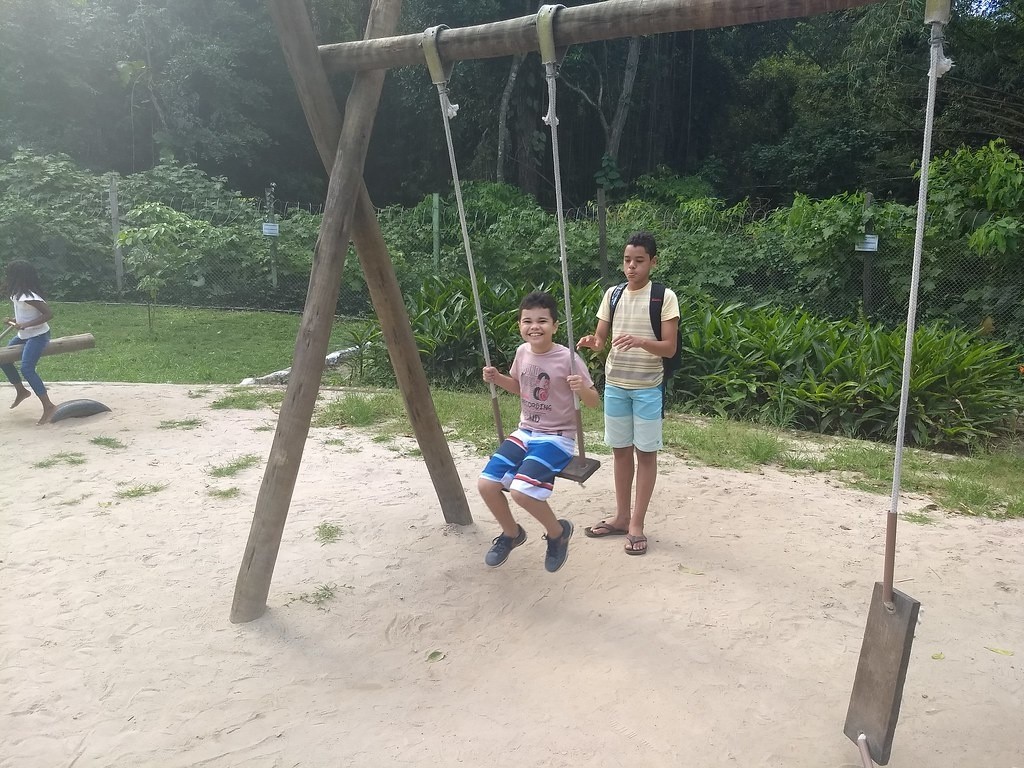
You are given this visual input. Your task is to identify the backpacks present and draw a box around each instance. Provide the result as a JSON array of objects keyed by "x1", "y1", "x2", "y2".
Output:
[{"x1": 609, "y1": 279, "x2": 681, "y2": 380}]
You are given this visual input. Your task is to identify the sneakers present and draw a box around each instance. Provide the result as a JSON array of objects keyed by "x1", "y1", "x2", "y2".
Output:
[
  {"x1": 485, "y1": 523, "x2": 527, "y2": 568},
  {"x1": 541, "y1": 519, "x2": 574, "y2": 573}
]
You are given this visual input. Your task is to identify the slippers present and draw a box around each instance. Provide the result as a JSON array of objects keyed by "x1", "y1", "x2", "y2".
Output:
[
  {"x1": 624, "y1": 533, "x2": 647, "y2": 554},
  {"x1": 585, "y1": 520, "x2": 629, "y2": 538}
]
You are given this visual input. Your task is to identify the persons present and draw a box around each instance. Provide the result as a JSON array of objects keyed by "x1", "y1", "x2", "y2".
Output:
[
  {"x1": 0, "y1": 260, "x2": 56, "y2": 425},
  {"x1": 575, "y1": 232, "x2": 680, "y2": 555},
  {"x1": 477, "y1": 293, "x2": 598, "y2": 572}
]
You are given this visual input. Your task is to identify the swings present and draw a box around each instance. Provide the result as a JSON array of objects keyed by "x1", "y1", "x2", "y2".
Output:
[{"x1": 435, "y1": 56, "x2": 601, "y2": 484}]
